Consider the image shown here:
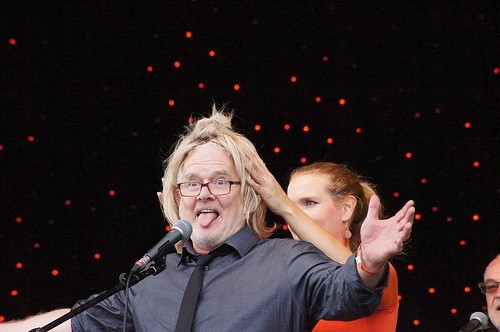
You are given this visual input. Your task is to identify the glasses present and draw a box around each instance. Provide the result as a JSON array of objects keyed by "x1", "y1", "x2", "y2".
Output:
[
  {"x1": 478, "y1": 282, "x2": 500, "y2": 293},
  {"x1": 176, "y1": 181, "x2": 240, "y2": 197}
]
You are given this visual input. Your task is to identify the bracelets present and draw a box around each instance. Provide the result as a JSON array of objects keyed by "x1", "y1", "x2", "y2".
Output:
[{"x1": 355, "y1": 244, "x2": 385, "y2": 274}]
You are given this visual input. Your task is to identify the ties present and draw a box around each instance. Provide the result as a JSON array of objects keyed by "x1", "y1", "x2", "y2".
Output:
[{"x1": 175, "y1": 244, "x2": 236, "y2": 332}]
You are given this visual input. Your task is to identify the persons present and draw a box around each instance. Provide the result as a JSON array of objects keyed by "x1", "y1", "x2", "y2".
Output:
[
  {"x1": 478, "y1": 255, "x2": 500, "y2": 332},
  {"x1": 157, "y1": 151, "x2": 398, "y2": 332},
  {"x1": 0, "y1": 104, "x2": 415, "y2": 332}
]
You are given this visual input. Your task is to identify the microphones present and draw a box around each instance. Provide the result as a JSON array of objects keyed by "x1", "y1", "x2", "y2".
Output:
[
  {"x1": 130, "y1": 219, "x2": 192, "y2": 273},
  {"x1": 461, "y1": 312, "x2": 489, "y2": 332}
]
